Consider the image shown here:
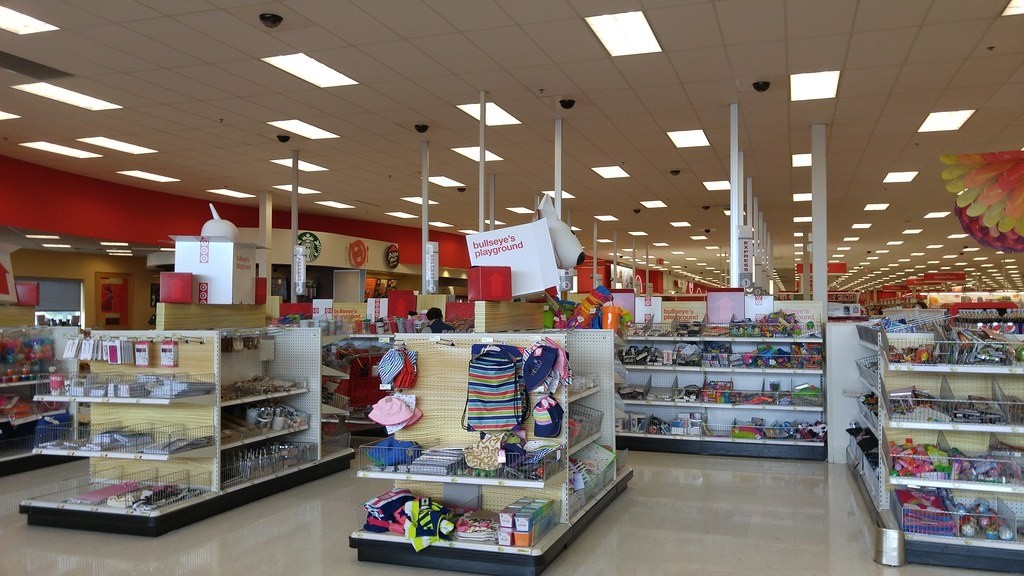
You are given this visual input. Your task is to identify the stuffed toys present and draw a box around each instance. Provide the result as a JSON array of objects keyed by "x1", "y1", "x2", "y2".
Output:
[{"x1": 526, "y1": 193, "x2": 585, "y2": 295}]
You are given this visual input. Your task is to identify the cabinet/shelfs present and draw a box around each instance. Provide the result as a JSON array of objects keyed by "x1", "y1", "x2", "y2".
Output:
[
  {"x1": 0, "y1": 327, "x2": 89, "y2": 478},
  {"x1": 19, "y1": 327, "x2": 355, "y2": 538},
  {"x1": 348, "y1": 329, "x2": 633, "y2": 576},
  {"x1": 614, "y1": 322, "x2": 826, "y2": 461},
  {"x1": 845, "y1": 324, "x2": 1024, "y2": 573}
]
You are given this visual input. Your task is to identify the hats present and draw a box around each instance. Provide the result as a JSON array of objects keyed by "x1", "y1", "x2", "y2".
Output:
[
  {"x1": 368, "y1": 393, "x2": 423, "y2": 435},
  {"x1": 532, "y1": 396, "x2": 564, "y2": 437},
  {"x1": 522, "y1": 346, "x2": 557, "y2": 393}
]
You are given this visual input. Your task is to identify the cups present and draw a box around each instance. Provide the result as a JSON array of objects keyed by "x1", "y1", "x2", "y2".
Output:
[
  {"x1": 50, "y1": 374, "x2": 63, "y2": 390},
  {"x1": 299, "y1": 319, "x2": 342, "y2": 337},
  {"x1": 388, "y1": 311, "x2": 428, "y2": 333},
  {"x1": 352, "y1": 317, "x2": 385, "y2": 335}
]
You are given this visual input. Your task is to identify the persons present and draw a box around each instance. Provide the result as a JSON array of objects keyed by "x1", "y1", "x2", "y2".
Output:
[
  {"x1": 426, "y1": 307, "x2": 455, "y2": 333},
  {"x1": 407, "y1": 311, "x2": 417, "y2": 321}
]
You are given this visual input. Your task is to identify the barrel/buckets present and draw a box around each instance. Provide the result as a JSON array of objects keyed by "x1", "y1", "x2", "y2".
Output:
[
  {"x1": 543, "y1": 303, "x2": 554, "y2": 328},
  {"x1": 246, "y1": 409, "x2": 286, "y2": 431},
  {"x1": 591, "y1": 306, "x2": 622, "y2": 329}
]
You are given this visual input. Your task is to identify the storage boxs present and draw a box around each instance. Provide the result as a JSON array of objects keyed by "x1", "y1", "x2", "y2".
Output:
[{"x1": 167, "y1": 235, "x2": 269, "y2": 305}]
